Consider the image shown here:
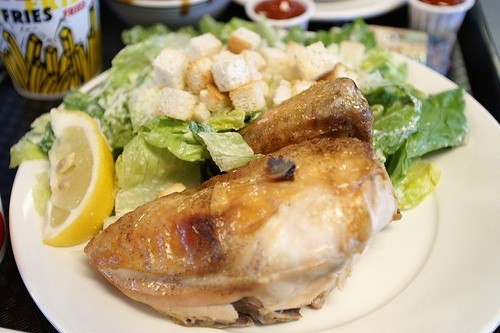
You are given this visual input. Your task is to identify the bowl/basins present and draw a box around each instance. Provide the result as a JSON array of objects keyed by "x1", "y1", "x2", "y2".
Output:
[
  {"x1": 109, "y1": 0, "x2": 232, "y2": 28},
  {"x1": 408, "y1": 0, "x2": 476, "y2": 38},
  {"x1": 245, "y1": 0, "x2": 315, "y2": 31}
]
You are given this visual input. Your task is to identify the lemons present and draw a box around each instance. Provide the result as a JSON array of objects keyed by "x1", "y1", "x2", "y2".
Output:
[{"x1": 42, "y1": 110, "x2": 117, "y2": 248}]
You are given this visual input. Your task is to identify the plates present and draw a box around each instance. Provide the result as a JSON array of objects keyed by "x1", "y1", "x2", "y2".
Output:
[
  {"x1": 232, "y1": 0, "x2": 408, "y2": 21},
  {"x1": 8, "y1": 48, "x2": 500, "y2": 333}
]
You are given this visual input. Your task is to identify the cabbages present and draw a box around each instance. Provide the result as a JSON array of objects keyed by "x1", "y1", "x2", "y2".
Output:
[{"x1": 10, "y1": 13, "x2": 470, "y2": 212}]
